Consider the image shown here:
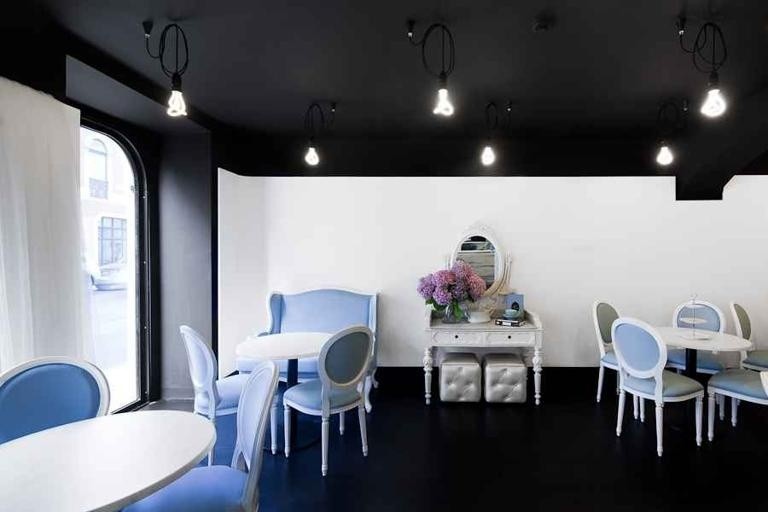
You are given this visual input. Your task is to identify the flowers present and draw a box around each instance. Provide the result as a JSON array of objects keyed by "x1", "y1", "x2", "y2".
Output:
[{"x1": 416, "y1": 257, "x2": 488, "y2": 307}]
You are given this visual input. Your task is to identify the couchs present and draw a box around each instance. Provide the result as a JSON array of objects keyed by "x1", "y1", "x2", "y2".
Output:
[{"x1": 239, "y1": 286, "x2": 382, "y2": 412}]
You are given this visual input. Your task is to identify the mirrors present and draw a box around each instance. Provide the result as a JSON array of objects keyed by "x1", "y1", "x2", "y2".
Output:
[{"x1": 448, "y1": 229, "x2": 511, "y2": 300}]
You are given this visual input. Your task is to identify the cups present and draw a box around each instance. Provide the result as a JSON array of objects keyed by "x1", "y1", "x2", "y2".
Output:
[{"x1": 505, "y1": 309, "x2": 520, "y2": 316}]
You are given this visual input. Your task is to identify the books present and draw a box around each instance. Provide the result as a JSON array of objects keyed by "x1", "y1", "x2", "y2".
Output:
[{"x1": 494, "y1": 317, "x2": 528, "y2": 327}]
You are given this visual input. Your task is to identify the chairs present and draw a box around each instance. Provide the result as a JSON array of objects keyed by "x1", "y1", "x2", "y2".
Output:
[
  {"x1": 591, "y1": 297, "x2": 768, "y2": 457},
  {"x1": 283, "y1": 327, "x2": 381, "y2": 475},
  {"x1": 119, "y1": 362, "x2": 282, "y2": 512},
  {"x1": 177, "y1": 324, "x2": 252, "y2": 465},
  {"x1": 2, "y1": 356, "x2": 111, "y2": 441}
]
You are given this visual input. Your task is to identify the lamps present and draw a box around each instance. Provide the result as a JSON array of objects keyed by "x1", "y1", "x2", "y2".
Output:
[
  {"x1": 651, "y1": 98, "x2": 686, "y2": 168},
  {"x1": 134, "y1": 21, "x2": 197, "y2": 121},
  {"x1": 406, "y1": 17, "x2": 459, "y2": 121},
  {"x1": 677, "y1": 13, "x2": 743, "y2": 122},
  {"x1": 294, "y1": 104, "x2": 338, "y2": 169},
  {"x1": 468, "y1": 97, "x2": 512, "y2": 176}
]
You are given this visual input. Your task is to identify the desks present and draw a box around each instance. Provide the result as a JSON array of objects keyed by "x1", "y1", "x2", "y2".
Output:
[
  {"x1": 5, "y1": 406, "x2": 217, "y2": 512},
  {"x1": 237, "y1": 325, "x2": 342, "y2": 452},
  {"x1": 425, "y1": 308, "x2": 547, "y2": 405}
]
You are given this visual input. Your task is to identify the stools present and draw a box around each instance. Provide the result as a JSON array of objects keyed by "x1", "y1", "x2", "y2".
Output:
[
  {"x1": 439, "y1": 351, "x2": 481, "y2": 402},
  {"x1": 483, "y1": 351, "x2": 526, "y2": 404}
]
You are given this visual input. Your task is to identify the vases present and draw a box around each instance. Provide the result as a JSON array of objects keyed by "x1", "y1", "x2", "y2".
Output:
[{"x1": 439, "y1": 301, "x2": 471, "y2": 326}]
